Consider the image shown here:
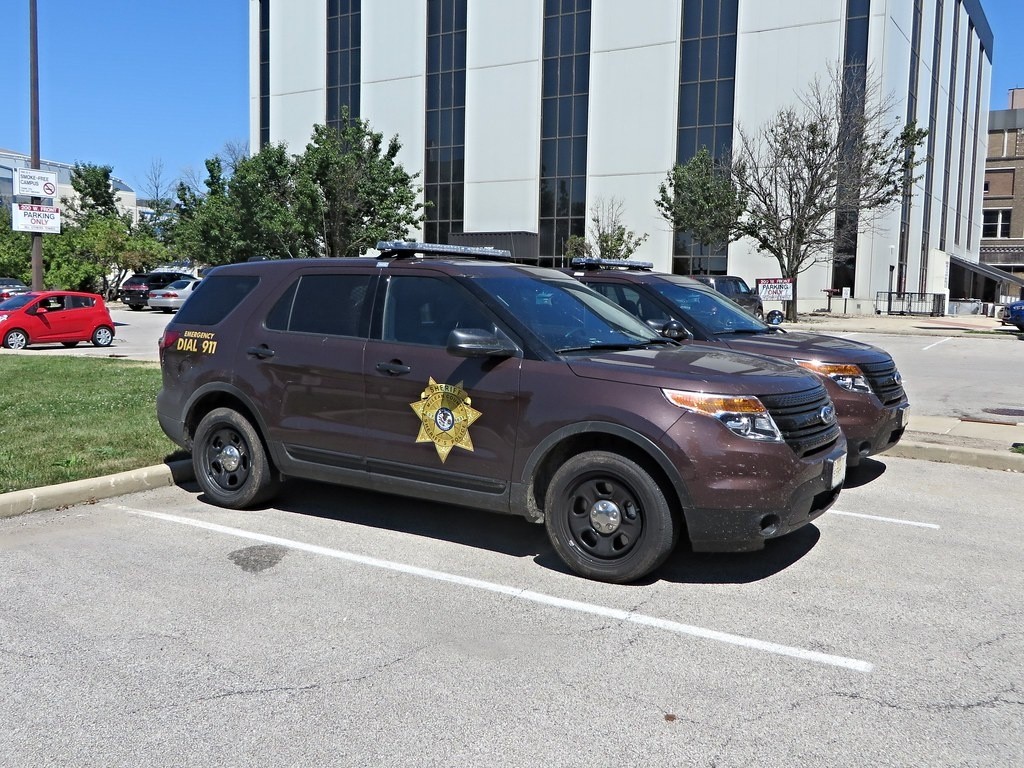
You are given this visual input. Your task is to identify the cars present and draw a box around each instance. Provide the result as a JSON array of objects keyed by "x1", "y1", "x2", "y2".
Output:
[
  {"x1": 0, "y1": 277, "x2": 32, "y2": 303},
  {"x1": 121, "y1": 272, "x2": 198, "y2": 310},
  {"x1": 0, "y1": 291, "x2": 115, "y2": 350},
  {"x1": 1003, "y1": 300, "x2": 1023, "y2": 332},
  {"x1": 148, "y1": 280, "x2": 202, "y2": 313}
]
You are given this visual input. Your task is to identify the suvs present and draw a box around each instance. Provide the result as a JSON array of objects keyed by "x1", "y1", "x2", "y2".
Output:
[
  {"x1": 555, "y1": 258, "x2": 911, "y2": 468},
  {"x1": 157, "y1": 243, "x2": 849, "y2": 583},
  {"x1": 686, "y1": 275, "x2": 764, "y2": 323}
]
